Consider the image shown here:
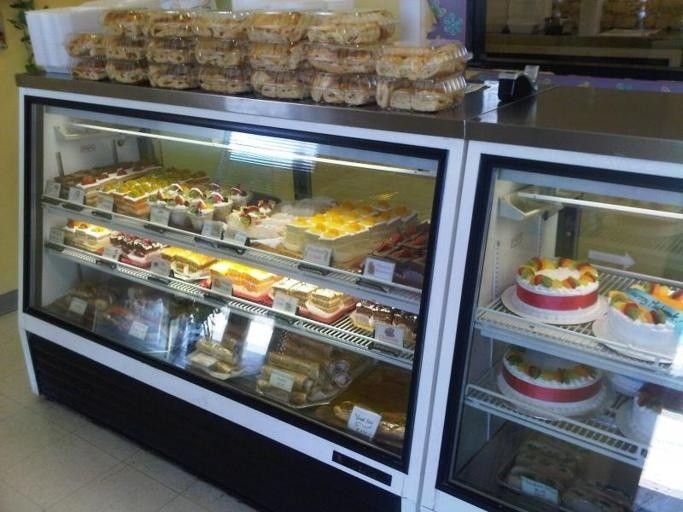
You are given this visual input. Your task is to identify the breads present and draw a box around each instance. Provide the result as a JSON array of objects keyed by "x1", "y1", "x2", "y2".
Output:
[
  {"x1": 65, "y1": 6, "x2": 473, "y2": 113},
  {"x1": 506, "y1": 427, "x2": 633, "y2": 512}
]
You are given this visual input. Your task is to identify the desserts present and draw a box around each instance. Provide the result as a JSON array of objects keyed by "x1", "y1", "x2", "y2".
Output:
[{"x1": 50, "y1": 159, "x2": 432, "y2": 437}]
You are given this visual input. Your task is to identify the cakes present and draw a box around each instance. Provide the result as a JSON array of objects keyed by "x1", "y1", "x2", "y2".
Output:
[{"x1": 502, "y1": 256, "x2": 683, "y2": 444}]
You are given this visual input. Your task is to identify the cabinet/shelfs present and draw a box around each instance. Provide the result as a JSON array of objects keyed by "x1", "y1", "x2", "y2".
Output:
[
  {"x1": 11, "y1": 69, "x2": 586, "y2": 512},
  {"x1": 419, "y1": 70, "x2": 683, "y2": 512}
]
